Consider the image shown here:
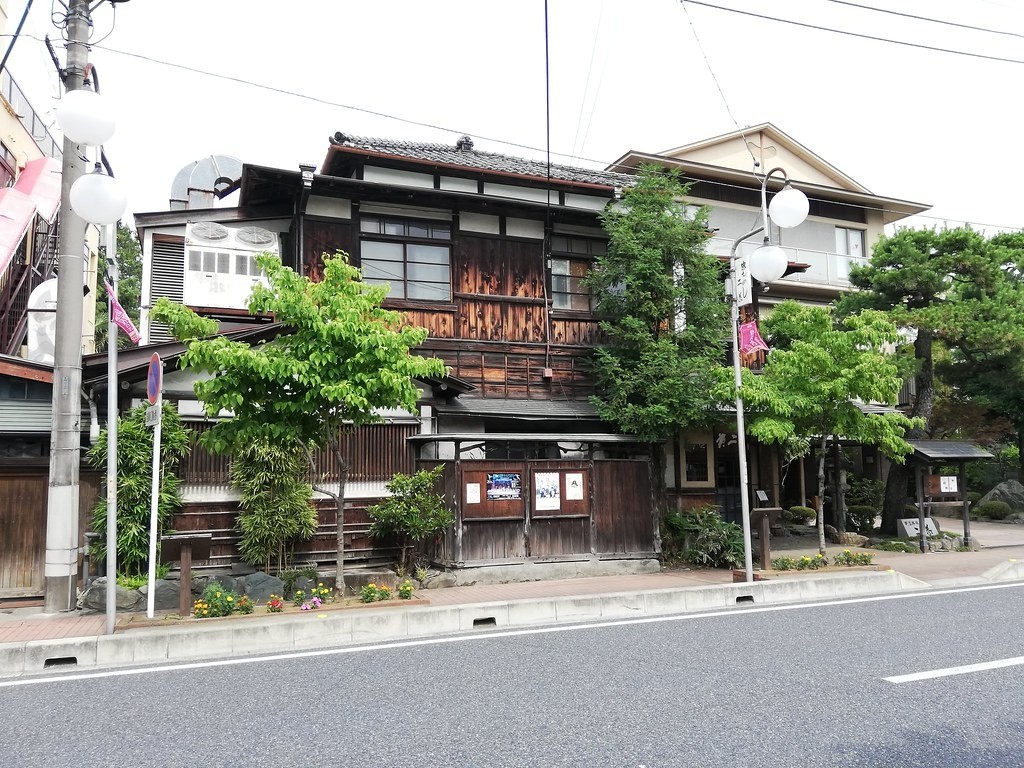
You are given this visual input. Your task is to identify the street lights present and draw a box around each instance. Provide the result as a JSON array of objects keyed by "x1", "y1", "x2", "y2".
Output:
[{"x1": 725, "y1": 166, "x2": 811, "y2": 582}]
